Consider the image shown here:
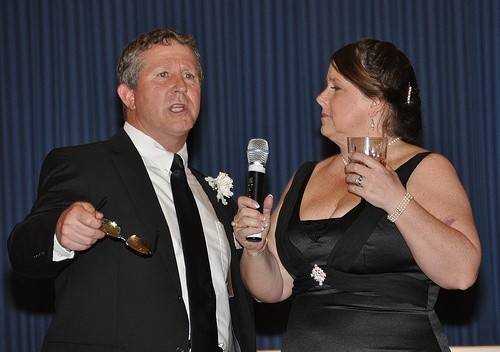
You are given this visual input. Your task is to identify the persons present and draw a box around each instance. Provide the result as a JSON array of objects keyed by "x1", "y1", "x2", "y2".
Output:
[
  {"x1": 7, "y1": 27, "x2": 275, "y2": 352},
  {"x1": 233, "y1": 39, "x2": 482, "y2": 352}
]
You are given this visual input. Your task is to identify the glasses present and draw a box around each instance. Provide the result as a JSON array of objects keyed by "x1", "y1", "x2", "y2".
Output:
[{"x1": 95, "y1": 196, "x2": 159, "y2": 255}]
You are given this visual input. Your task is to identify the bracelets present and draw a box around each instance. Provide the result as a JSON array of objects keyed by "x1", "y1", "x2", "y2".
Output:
[
  {"x1": 244, "y1": 238, "x2": 268, "y2": 257},
  {"x1": 387, "y1": 192, "x2": 414, "y2": 223}
]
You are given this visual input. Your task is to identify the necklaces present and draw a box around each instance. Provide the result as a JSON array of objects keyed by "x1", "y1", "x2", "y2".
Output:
[{"x1": 340, "y1": 136, "x2": 400, "y2": 166}]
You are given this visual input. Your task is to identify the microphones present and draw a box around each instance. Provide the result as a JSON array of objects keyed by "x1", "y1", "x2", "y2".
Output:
[{"x1": 245, "y1": 138, "x2": 269, "y2": 241}]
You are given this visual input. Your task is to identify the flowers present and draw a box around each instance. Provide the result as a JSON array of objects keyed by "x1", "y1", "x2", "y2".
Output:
[{"x1": 204, "y1": 171, "x2": 234, "y2": 205}]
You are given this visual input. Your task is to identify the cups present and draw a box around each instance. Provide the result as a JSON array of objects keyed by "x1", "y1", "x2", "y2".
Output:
[{"x1": 347, "y1": 137, "x2": 389, "y2": 178}]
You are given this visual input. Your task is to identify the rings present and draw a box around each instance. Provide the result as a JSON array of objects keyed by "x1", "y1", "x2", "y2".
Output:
[
  {"x1": 355, "y1": 176, "x2": 363, "y2": 187},
  {"x1": 233, "y1": 215, "x2": 239, "y2": 222},
  {"x1": 231, "y1": 221, "x2": 240, "y2": 229}
]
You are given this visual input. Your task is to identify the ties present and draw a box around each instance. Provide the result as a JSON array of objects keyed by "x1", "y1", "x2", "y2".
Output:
[{"x1": 170, "y1": 153, "x2": 219, "y2": 352}]
